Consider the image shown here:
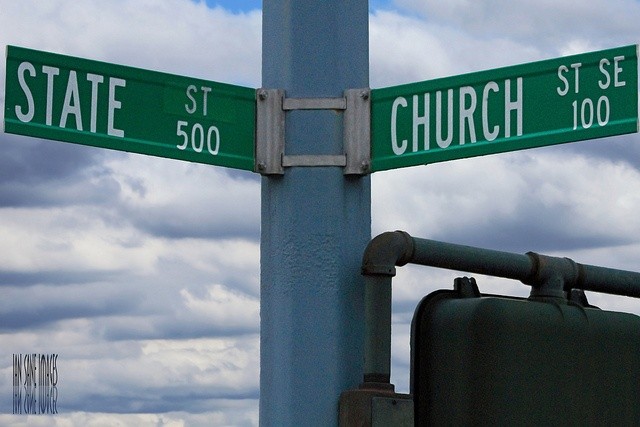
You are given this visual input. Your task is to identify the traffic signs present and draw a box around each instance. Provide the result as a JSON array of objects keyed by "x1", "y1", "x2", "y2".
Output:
[
  {"x1": 367, "y1": 44, "x2": 634, "y2": 172},
  {"x1": 4, "y1": 45, "x2": 254, "y2": 172}
]
